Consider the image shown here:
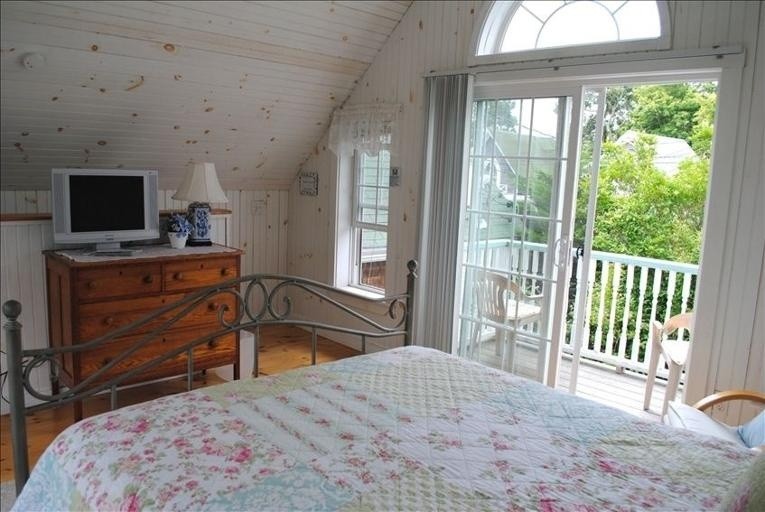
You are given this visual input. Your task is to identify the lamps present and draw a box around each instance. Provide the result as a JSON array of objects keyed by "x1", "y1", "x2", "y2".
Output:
[{"x1": 171, "y1": 161, "x2": 227, "y2": 247}]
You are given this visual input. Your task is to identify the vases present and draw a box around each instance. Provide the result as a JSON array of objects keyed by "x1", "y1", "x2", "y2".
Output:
[{"x1": 169, "y1": 232, "x2": 187, "y2": 249}]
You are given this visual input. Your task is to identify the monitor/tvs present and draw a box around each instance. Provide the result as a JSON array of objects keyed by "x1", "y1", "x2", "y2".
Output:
[{"x1": 52, "y1": 167, "x2": 160, "y2": 254}]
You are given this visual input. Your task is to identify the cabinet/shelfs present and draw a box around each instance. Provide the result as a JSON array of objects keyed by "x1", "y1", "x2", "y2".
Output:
[{"x1": 39, "y1": 245, "x2": 245, "y2": 423}]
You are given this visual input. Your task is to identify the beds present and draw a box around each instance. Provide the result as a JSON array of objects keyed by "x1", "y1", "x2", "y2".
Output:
[{"x1": 0, "y1": 256, "x2": 765, "y2": 512}]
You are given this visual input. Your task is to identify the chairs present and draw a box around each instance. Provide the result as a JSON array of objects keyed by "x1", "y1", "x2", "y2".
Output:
[
  {"x1": 663, "y1": 391, "x2": 765, "y2": 451},
  {"x1": 471, "y1": 267, "x2": 547, "y2": 376},
  {"x1": 644, "y1": 310, "x2": 695, "y2": 423}
]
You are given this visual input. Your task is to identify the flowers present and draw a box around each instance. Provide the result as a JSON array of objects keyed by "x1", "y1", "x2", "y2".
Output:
[{"x1": 166, "y1": 214, "x2": 194, "y2": 233}]
